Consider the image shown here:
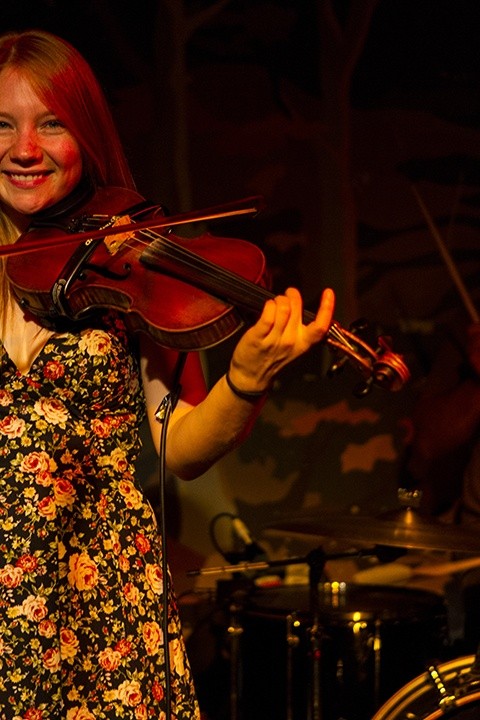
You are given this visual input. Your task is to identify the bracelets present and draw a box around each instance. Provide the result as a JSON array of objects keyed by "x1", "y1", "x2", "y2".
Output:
[{"x1": 226, "y1": 371, "x2": 275, "y2": 403}]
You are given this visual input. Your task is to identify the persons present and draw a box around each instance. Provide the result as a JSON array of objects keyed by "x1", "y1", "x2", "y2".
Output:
[{"x1": 0, "y1": 32, "x2": 335, "y2": 720}]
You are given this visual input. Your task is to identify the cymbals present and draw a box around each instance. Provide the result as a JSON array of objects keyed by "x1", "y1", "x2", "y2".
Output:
[{"x1": 257, "y1": 509, "x2": 480, "y2": 557}]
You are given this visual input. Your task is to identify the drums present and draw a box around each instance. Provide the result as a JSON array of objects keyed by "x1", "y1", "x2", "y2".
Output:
[
  {"x1": 373, "y1": 651, "x2": 480, "y2": 720},
  {"x1": 222, "y1": 579, "x2": 450, "y2": 720}
]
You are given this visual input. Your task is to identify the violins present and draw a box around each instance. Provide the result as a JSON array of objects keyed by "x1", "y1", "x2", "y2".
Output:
[{"x1": 0, "y1": 184, "x2": 414, "y2": 400}]
